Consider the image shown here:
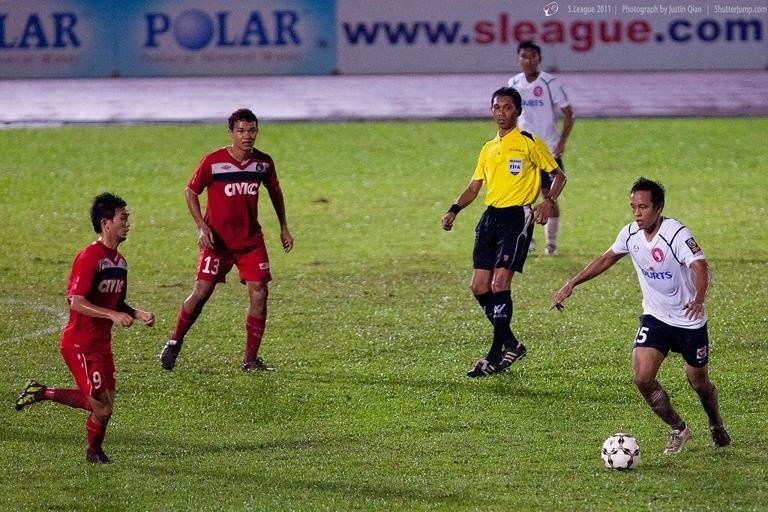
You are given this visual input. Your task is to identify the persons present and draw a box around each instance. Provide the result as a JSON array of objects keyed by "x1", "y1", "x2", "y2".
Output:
[
  {"x1": 13, "y1": 191, "x2": 157, "y2": 469},
  {"x1": 503, "y1": 35, "x2": 579, "y2": 257},
  {"x1": 439, "y1": 86, "x2": 564, "y2": 379},
  {"x1": 545, "y1": 173, "x2": 731, "y2": 456},
  {"x1": 158, "y1": 106, "x2": 293, "y2": 377}
]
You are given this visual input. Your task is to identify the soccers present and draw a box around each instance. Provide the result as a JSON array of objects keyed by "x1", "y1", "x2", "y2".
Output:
[{"x1": 601, "y1": 433, "x2": 640, "y2": 470}]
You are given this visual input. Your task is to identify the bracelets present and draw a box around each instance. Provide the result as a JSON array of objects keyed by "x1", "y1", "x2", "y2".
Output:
[
  {"x1": 540, "y1": 195, "x2": 555, "y2": 206},
  {"x1": 445, "y1": 203, "x2": 461, "y2": 216}
]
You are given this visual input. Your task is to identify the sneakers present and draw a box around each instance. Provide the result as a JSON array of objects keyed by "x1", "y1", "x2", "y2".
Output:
[
  {"x1": 528, "y1": 237, "x2": 559, "y2": 257},
  {"x1": 466, "y1": 341, "x2": 527, "y2": 379},
  {"x1": 708, "y1": 419, "x2": 731, "y2": 450},
  {"x1": 86, "y1": 448, "x2": 109, "y2": 464},
  {"x1": 160, "y1": 335, "x2": 183, "y2": 370},
  {"x1": 241, "y1": 357, "x2": 275, "y2": 372},
  {"x1": 662, "y1": 422, "x2": 691, "y2": 457},
  {"x1": 16, "y1": 380, "x2": 46, "y2": 410}
]
